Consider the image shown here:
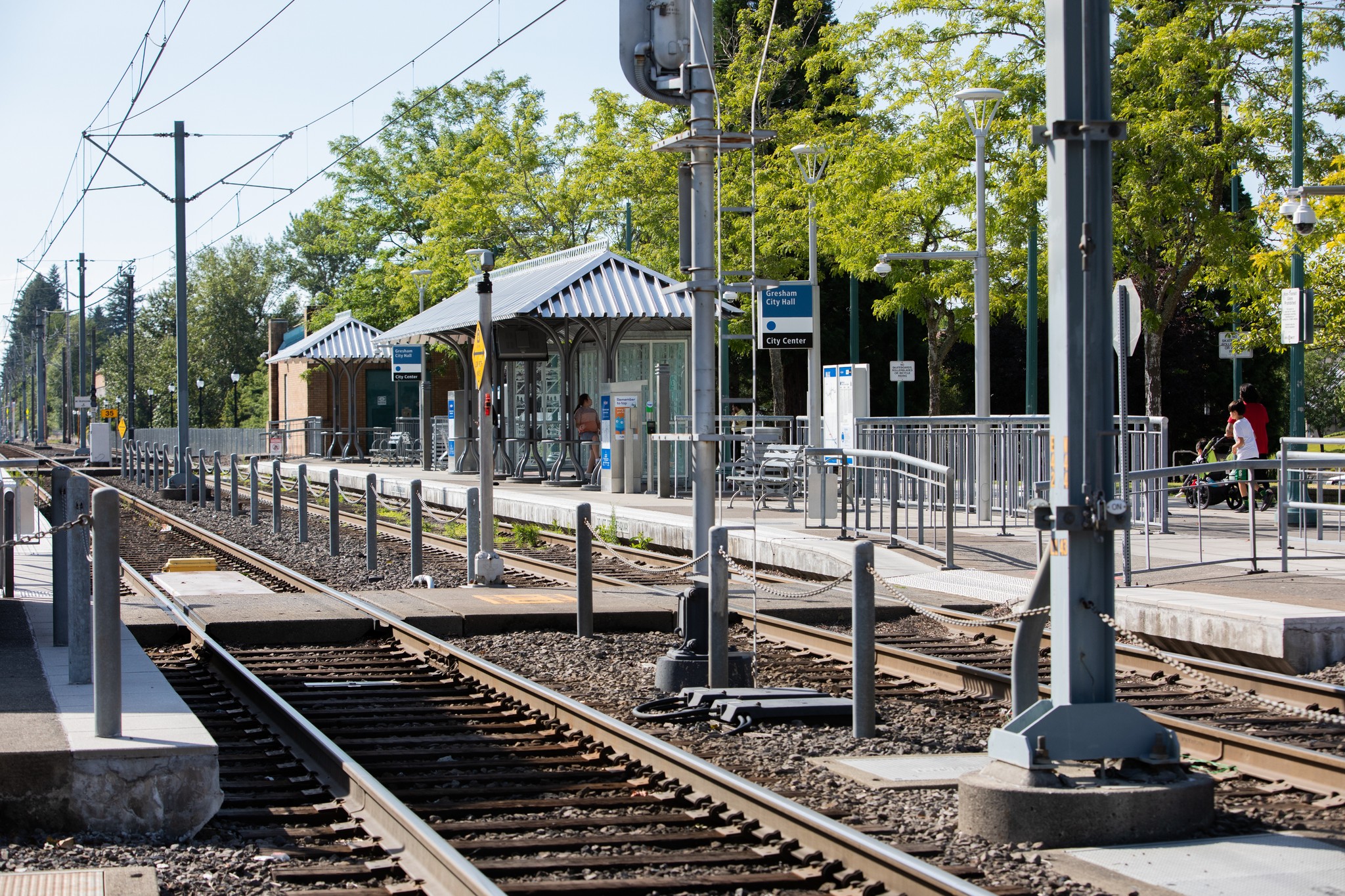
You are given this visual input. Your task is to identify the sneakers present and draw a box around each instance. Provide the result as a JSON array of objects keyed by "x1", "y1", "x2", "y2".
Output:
[
  {"x1": 1265, "y1": 488, "x2": 1277, "y2": 509},
  {"x1": 1260, "y1": 491, "x2": 1275, "y2": 512},
  {"x1": 1233, "y1": 503, "x2": 1249, "y2": 513},
  {"x1": 1175, "y1": 490, "x2": 1184, "y2": 498},
  {"x1": 1254, "y1": 500, "x2": 1259, "y2": 511},
  {"x1": 585, "y1": 474, "x2": 597, "y2": 482},
  {"x1": 596, "y1": 461, "x2": 600, "y2": 467}
]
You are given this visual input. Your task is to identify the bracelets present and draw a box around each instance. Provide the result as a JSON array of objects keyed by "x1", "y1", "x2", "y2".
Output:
[{"x1": 580, "y1": 425, "x2": 584, "y2": 431}]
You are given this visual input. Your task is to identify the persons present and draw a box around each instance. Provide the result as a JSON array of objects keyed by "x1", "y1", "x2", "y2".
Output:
[
  {"x1": 1228, "y1": 399, "x2": 1275, "y2": 513},
  {"x1": 473, "y1": 404, "x2": 498, "y2": 481},
  {"x1": 1224, "y1": 383, "x2": 1278, "y2": 511},
  {"x1": 729, "y1": 403, "x2": 747, "y2": 462},
  {"x1": 1175, "y1": 438, "x2": 1211, "y2": 498},
  {"x1": 574, "y1": 394, "x2": 600, "y2": 482}
]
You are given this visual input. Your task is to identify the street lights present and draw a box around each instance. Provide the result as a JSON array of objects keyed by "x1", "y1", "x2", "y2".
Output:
[
  {"x1": 147, "y1": 386, "x2": 154, "y2": 428},
  {"x1": 133, "y1": 392, "x2": 136, "y2": 400},
  {"x1": 874, "y1": 86, "x2": 1007, "y2": 522},
  {"x1": 167, "y1": 382, "x2": 176, "y2": 428},
  {"x1": 115, "y1": 395, "x2": 122, "y2": 430},
  {"x1": 87, "y1": 410, "x2": 91, "y2": 433},
  {"x1": 464, "y1": 245, "x2": 506, "y2": 588},
  {"x1": 103, "y1": 398, "x2": 108, "y2": 422},
  {"x1": 195, "y1": 377, "x2": 206, "y2": 429},
  {"x1": 409, "y1": 268, "x2": 438, "y2": 472},
  {"x1": 230, "y1": 368, "x2": 241, "y2": 427},
  {"x1": 788, "y1": 143, "x2": 831, "y2": 453}
]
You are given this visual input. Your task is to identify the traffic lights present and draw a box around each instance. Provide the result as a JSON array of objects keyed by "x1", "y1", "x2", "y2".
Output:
[{"x1": 90, "y1": 384, "x2": 97, "y2": 407}]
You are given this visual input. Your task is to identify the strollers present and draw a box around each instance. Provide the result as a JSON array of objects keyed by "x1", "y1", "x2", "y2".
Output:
[{"x1": 1185, "y1": 435, "x2": 1246, "y2": 510}]
